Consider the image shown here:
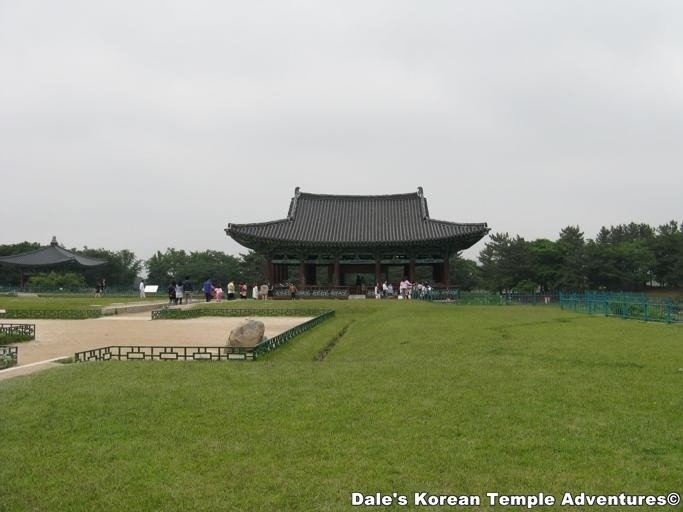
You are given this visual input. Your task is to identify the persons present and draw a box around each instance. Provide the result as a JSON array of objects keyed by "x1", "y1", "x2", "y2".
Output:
[
  {"x1": 251, "y1": 279, "x2": 275, "y2": 303},
  {"x1": 167, "y1": 281, "x2": 175, "y2": 305},
  {"x1": 227, "y1": 278, "x2": 235, "y2": 299},
  {"x1": 182, "y1": 276, "x2": 193, "y2": 304},
  {"x1": 213, "y1": 285, "x2": 222, "y2": 302},
  {"x1": 99, "y1": 277, "x2": 106, "y2": 296},
  {"x1": 354, "y1": 274, "x2": 432, "y2": 299},
  {"x1": 280, "y1": 279, "x2": 287, "y2": 287},
  {"x1": 93, "y1": 280, "x2": 102, "y2": 298},
  {"x1": 238, "y1": 282, "x2": 247, "y2": 292},
  {"x1": 286, "y1": 281, "x2": 296, "y2": 301},
  {"x1": 201, "y1": 277, "x2": 215, "y2": 302},
  {"x1": 238, "y1": 282, "x2": 248, "y2": 299},
  {"x1": 174, "y1": 280, "x2": 184, "y2": 305},
  {"x1": 137, "y1": 279, "x2": 146, "y2": 301}
]
